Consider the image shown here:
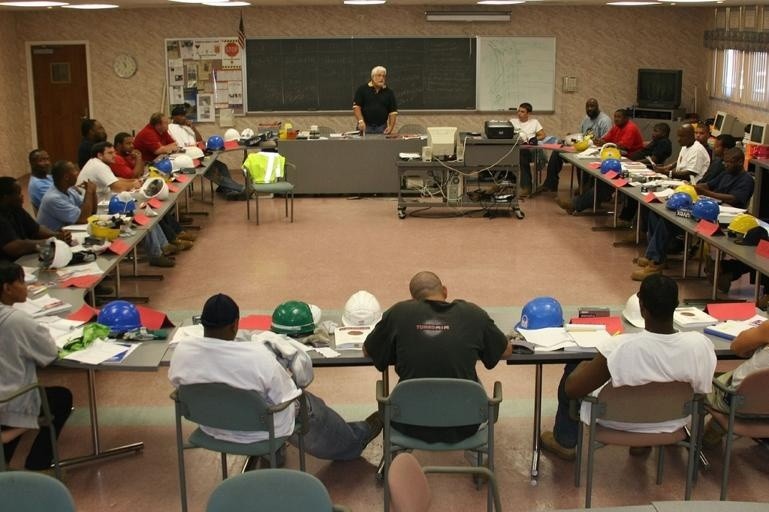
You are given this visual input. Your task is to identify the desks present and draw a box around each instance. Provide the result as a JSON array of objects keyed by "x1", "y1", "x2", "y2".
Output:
[
  {"x1": 521, "y1": 139, "x2": 769, "y2": 305},
  {"x1": 3, "y1": 135, "x2": 222, "y2": 305},
  {"x1": 244, "y1": 129, "x2": 430, "y2": 199}
]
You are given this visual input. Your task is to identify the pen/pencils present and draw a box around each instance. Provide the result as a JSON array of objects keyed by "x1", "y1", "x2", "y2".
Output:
[
  {"x1": 116, "y1": 344, "x2": 131, "y2": 348},
  {"x1": 612, "y1": 331, "x2": 621, "y2": 338},
  {"x1": 307, "y1": 339, "x2": 318, "y2": 349},
  {"x1": 34, "y1": 287, "x2": 47, "y2": 296}
]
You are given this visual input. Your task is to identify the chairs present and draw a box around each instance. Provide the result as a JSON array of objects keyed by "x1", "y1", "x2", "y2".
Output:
[
  {"x1": 398, "y1": 123, "x2": 428, "y2": 138},
  {"x1": 245, "y1": 151, "x2": 299, "y2": 225},
  {"x1": 2, "y1": 305, "x2": 769, "y2": 512}
]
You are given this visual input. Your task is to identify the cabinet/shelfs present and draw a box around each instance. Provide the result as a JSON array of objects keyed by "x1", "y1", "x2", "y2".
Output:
[{"x1": 627, "y1": 106, "x2": 689, "y2": 121}]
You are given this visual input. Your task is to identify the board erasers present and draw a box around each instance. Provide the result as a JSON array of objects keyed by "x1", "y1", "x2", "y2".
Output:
[{"x1": 509, "y1": 108, "x2": 517, "y2": 110}]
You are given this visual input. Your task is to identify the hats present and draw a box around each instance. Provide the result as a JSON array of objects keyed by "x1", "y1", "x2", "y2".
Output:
[{"x1": 735, "y1": 226, "x2": 768, "y2": 246}]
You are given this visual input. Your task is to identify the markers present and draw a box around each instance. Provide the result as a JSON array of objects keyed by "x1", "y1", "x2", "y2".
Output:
[{"x1": 296, "y1": 137, "x2": 307, "y2": 139}]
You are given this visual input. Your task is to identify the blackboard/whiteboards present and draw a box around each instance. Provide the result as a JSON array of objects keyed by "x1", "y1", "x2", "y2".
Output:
[{"x1": 246, "y1": 34, "x2": 557, "y2": 115}]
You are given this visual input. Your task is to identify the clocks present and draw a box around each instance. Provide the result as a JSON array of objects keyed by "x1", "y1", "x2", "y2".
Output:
[{"x1": 114, "y1": 53, "x2": 138, "y2": 79}]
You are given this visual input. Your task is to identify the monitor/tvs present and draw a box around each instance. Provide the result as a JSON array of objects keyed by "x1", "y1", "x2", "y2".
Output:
[{"x1": 639, "y1": 68, "x2": 683, "y2": 108}]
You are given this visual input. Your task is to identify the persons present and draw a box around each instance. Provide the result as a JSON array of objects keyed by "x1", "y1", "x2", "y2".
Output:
[{"x1": 354, "y1": 67, "x2": 397, "y2": 135}]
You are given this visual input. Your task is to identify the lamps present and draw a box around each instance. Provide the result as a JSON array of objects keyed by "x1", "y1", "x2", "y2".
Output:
[{"x1": 421, "y1": 9, "x2": 514, "y2": 23}]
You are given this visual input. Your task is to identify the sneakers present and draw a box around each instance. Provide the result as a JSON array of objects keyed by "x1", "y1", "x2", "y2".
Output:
[
  {"x1": 550, "y1": 196, "x2": 574, "y2": 215},
  {"x1": 631, "y1": 251, "x2": 652, "y2": 266},
  {"x1": 174, "y1": 230, "x2": 197, "y2": 241},
  {"x1": 149, "y1": 256, "x2": 176, "y2": 268},
  {"x1": 360, "y1": 411, "x2": 386, "y2": 453},
  {"x1": 702, "y1": 260, "x2": 723, "y2": 277},
  {"x1": 159, "y1": 242, "x2": 179, "y2": 255},
  {"x1": 170, "y1": 239, "x2": 193, "y2": 250},
  {"x1": 94, "y1": 286, "x2": 113, "y2": 295},
  {"x1": 706, "y1": 271, "x2": 733, "y2": 295},
  {"x1": 541, "y1": 429, "x2": 577, "y2": 462},
  {"x1": 223, "y1": 187, "x2": 256, "y2": 201},
  {"x1": 630, "y1": 262, "x2": 664, "y2": 282},
  {"x1": 518, "y1": 184, "x2": 559, "y2": 199}
]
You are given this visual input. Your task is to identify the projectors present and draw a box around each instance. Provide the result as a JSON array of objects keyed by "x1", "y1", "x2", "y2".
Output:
[{"x1": 485, "y1": 119, "x2": 515, "y2": 139}]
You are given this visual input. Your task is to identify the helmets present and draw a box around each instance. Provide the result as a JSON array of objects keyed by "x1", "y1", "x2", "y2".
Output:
[
  {"x1": 690, "y1": 197, "x2": 721, "y2": 223},
  {"x1": 600, "y1": 158, "x2": 623, "y2": 176},
  {"x1": 269, "y1": 301, "x2": 316, "y2": 340},
  {"x1": 572, "y1": 138, "x2": 589, "y2": 151},
  {"x1": 155, "y1": 156, "x2": 173, "y2": 176},
  {"x1": 340, "y1": 288, "x2": 384, "y2": 330},
  {"x1": 107, "y1": 192, "x2": 138, "y2": 218},
  {"x1": 204, "y1": 136, "x2": 225, "y2": 153},
  {"x1": 97, "y1": 299, "x2": 144, "y2": 340},
  {"x1": 665, "y1": 192, "x2": 693, "y2": 213},
  {"x1": 166, "y1": 152, "x2": 195, "y2": 171},
  {"x1": 240, "y1": 128, "x2": 255, "y2": 140},
  {"x1": 223, "y1": 127, "x2": 241, "y2": 143},
  {"x1": 622, "y1": 292, "x2": 652, "y2": 329},
  {"x1": 85, "y1": 212, "x2": 126, "y2": 241},
  {"x1": 675, "y1": 183, "x2": 699, "y2": 205},
  {"x1": 139, "y1": 177, "x2": 170, "y2": 200},
  {"x1": 513, "y1": 295, "x2": 565, "y2": 336},
  {"x1": 601, "y1": 146, "x2": 621, "y2": 164},
  {"x1": 147, "y1": 165, "x2": 169, "y2": 184},
  {"x1": 728, "y1": 213, "x2": 759, "y2": 236},
  {"x1": 182, "y1": 144, "x2": 206, "y2": 162},
  {"x1": 40, "y1": 236, "x2": 74, "y2": 270}
]
